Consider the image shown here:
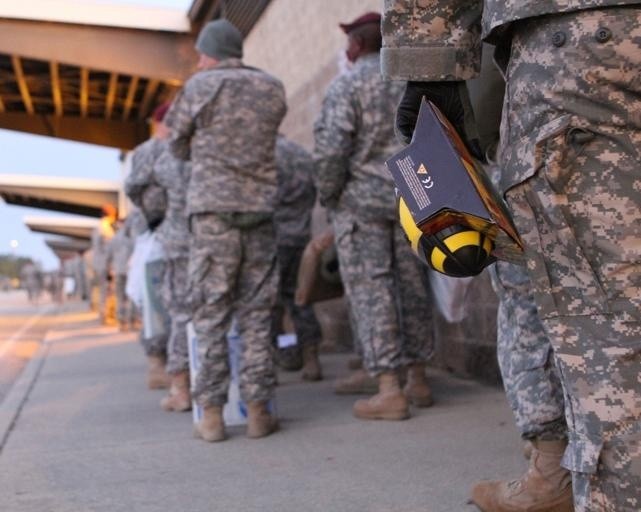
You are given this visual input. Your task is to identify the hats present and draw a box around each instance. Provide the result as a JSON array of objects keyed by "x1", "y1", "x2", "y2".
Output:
[
  {"x1": 194, "y1": 18, "x2": 244, "y2": 60},
  {"x1": 154, "y1": 103, "x2": 168, "y2": 121},
  {"x1": 340, "y1": 12, "x2": 380, "y2": 33}
]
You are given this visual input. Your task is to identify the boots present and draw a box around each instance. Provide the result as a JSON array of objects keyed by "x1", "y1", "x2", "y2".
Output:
[
  {"x1": 248, "y1": 398, "x2": 278, "y2": 437},
  {"x1": 472, "y1": 438, "x2": 574, "y2": 512},
  {"x1": 334, "y1": 355, "x2": 433, "y2": 420},
  {"x1": 147, "y1": 355, "x2": 226, "y2": 442},
  {"x1": 301, "y1": 345, "x2": 322, "y2": 378}
]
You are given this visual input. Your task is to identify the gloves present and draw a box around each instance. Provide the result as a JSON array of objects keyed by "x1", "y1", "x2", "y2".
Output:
[{"x1": 394, "y1": 80, "x2": 493, "y2": 163}]
